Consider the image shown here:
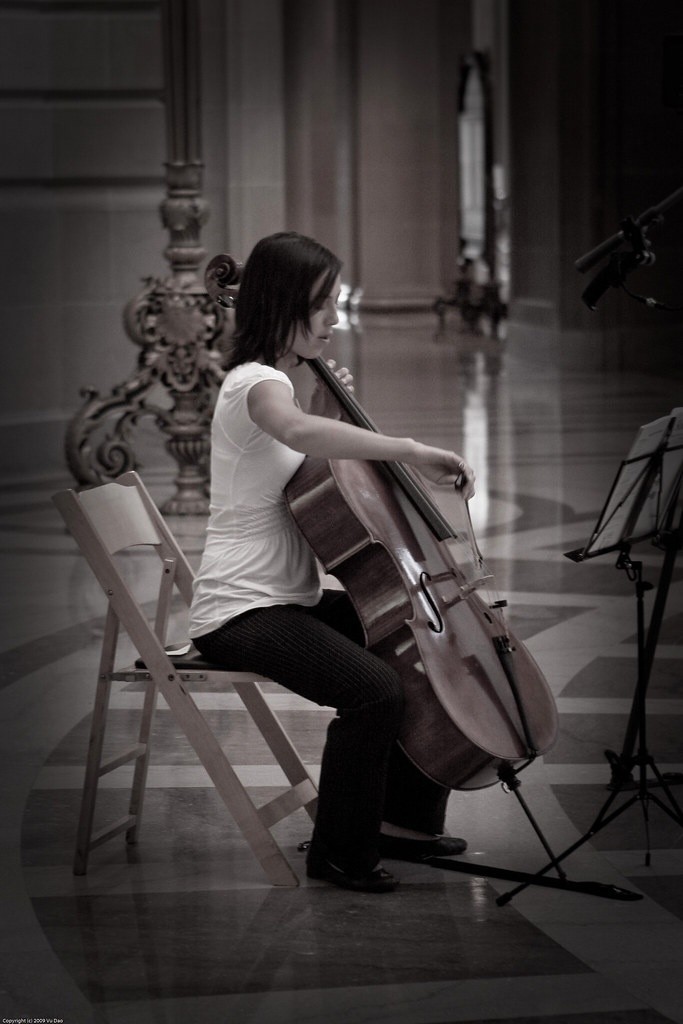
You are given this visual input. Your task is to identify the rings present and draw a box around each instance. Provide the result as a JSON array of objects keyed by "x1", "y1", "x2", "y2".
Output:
[{"x1": 457, "y1": 462, "x2": 465, "y2": 470}]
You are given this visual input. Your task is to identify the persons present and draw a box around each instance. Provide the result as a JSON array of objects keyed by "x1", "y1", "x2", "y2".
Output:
[{"x1": 187, "y1": 232, "x2": 477, "y2": 892}]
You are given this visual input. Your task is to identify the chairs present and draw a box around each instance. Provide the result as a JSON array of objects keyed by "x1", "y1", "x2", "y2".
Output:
[{"x1": 52, "y1": 472, "x2": 319, "y2": 887}]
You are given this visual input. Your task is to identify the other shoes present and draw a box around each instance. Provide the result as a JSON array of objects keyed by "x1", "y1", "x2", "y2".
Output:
[
  {"x1": 379, "y1": 832, "x2": 467, "y2": 855},
  {"x1": 306, "y1": 844, "x2": 399, "y2": 893}
]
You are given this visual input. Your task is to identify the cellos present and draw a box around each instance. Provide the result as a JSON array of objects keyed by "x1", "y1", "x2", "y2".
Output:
[{"x1": 200, "y1": 252, "x2": 566, "y2": 883}]
